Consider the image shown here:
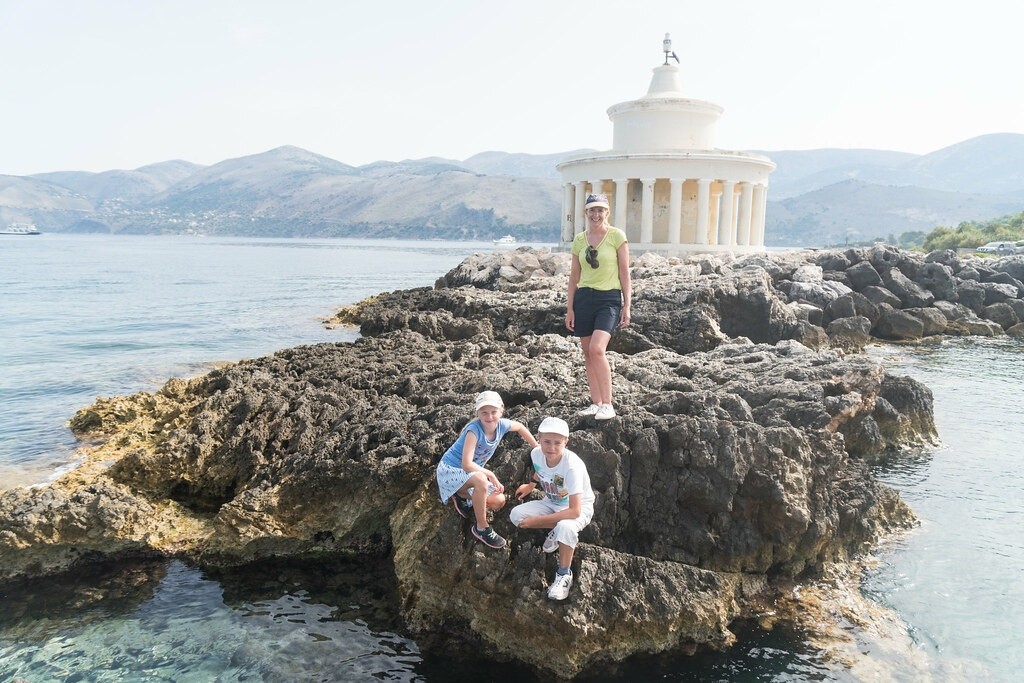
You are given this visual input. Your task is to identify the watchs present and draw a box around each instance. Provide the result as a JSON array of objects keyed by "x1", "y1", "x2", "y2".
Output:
[{"x1": 529, "y1": 476, "x2": 539, "y2": 484}]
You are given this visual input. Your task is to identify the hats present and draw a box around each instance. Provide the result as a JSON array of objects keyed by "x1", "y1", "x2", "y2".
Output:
[
  {"x1": 475, "y1": 391, "x2": 503, "y2": 409},
  {"x1": 584, "y1": 194, "x2": 610, "y2": 209},
  {"x1": 538, "y1": 417, "x2": 569, "y2": 438}
]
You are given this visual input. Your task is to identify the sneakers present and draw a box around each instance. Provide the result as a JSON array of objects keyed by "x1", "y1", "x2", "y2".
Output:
[
  {"x1": 595, "y1": 404, "x2": 616, "y2": 420},
  {"x1": 547, "y1": 570, "x2": 574, "y2": 601},
  {"x1": 579, "y1": 404, "x2": 600, "y2": 417},
  {"x1": 451, "y1": 493, "x2": 470, "y2": 519},
  {"x1": 471, "y1": 523, "x2": 506, "y2": 548},
  {"x1": 543, "y1": 527, "x2": 560, "y2": 554}
]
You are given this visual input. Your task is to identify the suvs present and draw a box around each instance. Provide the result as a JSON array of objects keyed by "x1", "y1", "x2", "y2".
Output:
[
  {"x1": 977, "y1": 242, "x2": 1017, "y2": 256},
  {"x1": 1014, "y1": 240, "x2": 1024, "y2": 254}
]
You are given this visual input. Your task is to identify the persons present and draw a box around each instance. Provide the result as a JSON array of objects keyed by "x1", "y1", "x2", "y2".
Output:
[
  {"x1": 435, "y1": 391, "x2": 542, "y2": 549},
  {"x1": 508, "y1": 417, "x2": 596, "y2": 600},
  {"x1": 564, "y1": 193, "x2": 632, "y2": 421}
]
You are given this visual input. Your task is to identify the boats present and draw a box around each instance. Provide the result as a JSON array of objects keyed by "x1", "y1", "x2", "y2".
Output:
[
  {"x1": 493, "y1": 235, "x2": 516, "y2": 246},
  {"x1": 0, "y1": 222, "x2": 42, "y2": 236}
]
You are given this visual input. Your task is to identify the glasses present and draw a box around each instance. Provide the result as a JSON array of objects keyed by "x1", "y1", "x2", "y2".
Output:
[
  {"x1": 590, "y1": 249, "x2": 599, "y2": 269},
  {"x1": 585, "y1": 245, "x2": 594, "y2": 264}
]
